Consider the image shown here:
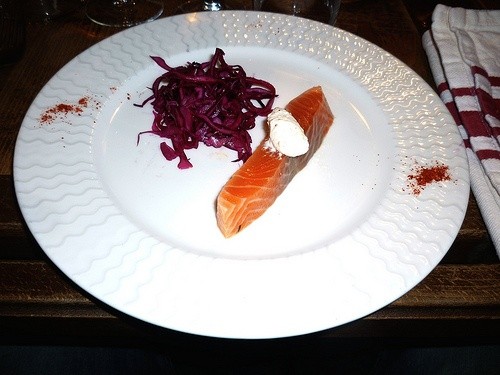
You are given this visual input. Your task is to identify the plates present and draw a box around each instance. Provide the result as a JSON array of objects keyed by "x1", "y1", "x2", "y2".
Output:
[{"x1": 15, "y1": 7, "x2": 471, "y2": 340}]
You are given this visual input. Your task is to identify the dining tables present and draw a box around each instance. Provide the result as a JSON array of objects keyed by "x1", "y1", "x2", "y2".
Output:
[{"x1": 0, "y1": 0, "x2": 499, "y2": 322}]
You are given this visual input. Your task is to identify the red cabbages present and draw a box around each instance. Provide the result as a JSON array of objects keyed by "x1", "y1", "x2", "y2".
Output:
[{"x1": 133, "y1": 46, "x2": 278, "y2": 169}]
